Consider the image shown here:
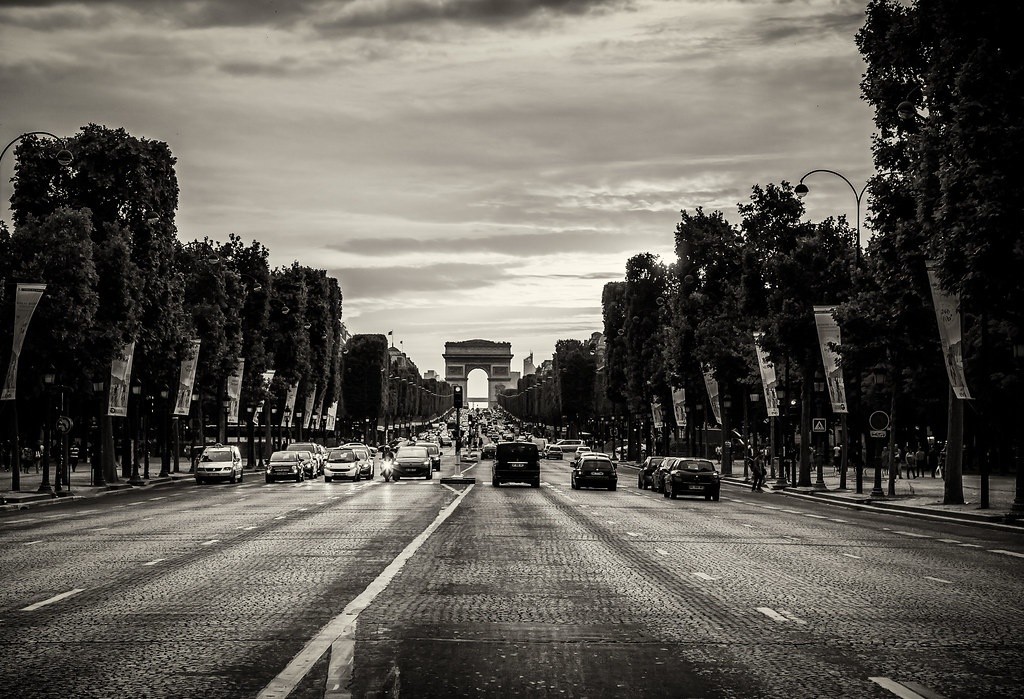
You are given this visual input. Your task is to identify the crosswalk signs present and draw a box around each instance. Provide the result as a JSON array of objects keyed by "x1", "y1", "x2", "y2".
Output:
[{"x1": 812, "y1": 418, "x2": 826, "y2": 433}]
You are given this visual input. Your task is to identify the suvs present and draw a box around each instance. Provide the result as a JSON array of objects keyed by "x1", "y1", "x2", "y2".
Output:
[
  {"x1": 322, "y1": 448, "x2": 362, "y2": 482},
  {"x1": 651, "y1": 456, "x2": 689, "y2": 493},
  {"x1": 636, "y1": 455, "x2": 668, "y2": 490},
  {"x1": 491, "y1": 441, "x2": 541, "y2": 489}
]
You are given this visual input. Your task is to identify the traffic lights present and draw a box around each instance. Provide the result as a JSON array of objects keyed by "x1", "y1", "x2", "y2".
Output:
[
  {"x1": 468, "y1": 415, "x2": 473, "y2": 426},
  {"x1": 453, "y1": 385, "x2": 463, "y2": 408}
]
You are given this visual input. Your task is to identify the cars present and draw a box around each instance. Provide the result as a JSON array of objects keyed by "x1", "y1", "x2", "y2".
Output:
[
  {"x1": 264, "y1": 451, "x2": 306, "y2": 483},
  {"x1": 662, "y1": 458, "x2": 722, "y2": 501},
  {"x1": 392, "y1": 445, "x2": 433, "y2": 481},
  {"x1": 570, "y1": 455, "x2": 618, "y2": 492},
  {"x1": 414, "y1": 443, "x2": 443, "y2": 471},
  {"x1": 285, "y1": 404, "x2": 651, "y2": 479}
]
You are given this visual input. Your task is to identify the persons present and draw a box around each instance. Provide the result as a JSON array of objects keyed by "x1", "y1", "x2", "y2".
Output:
[
  {"x1": 184, "y1": 444, "x2": 193, "y2": 462},
  {"x1": 382, "y1": 445, "x2": 393, "y2": 460},
  {"x1": 747, "y1": 443, "x2": 947, "y2": 481},
  {"x1": 70, "y1": 441, "x2": 80, "y2": 473},
  {"x1": 750, "y1": 452, "x2": 766, "y2": 493},
  {"x1": 715, "y1": 444, "x2": 723, "y2": 464},
  {"x1": 4, "y1": 439, "x2": 47, "y2": 474}
]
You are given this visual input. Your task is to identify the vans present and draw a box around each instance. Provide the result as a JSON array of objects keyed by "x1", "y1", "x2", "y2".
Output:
[{"x1": 194, "y1": 443, "x2": 244, "y2": 484}]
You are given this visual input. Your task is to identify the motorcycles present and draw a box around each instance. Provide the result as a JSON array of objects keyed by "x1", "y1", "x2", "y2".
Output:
[{"x1": 379, "y1": 456, "x2": 396, "y2": 482}]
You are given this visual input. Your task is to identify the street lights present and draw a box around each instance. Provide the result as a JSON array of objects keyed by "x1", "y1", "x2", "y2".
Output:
[
  {"x1": 794, "y1": 169, "x2": 911, "y2": 271},
  {"x1": 283, "y1": 403, "x2": 291, "y2": 450}
]
